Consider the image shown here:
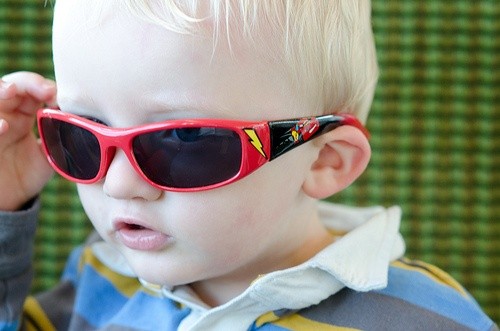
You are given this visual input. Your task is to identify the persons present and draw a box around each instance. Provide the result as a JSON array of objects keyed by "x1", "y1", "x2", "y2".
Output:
[{"x1": 0, "y1": 0, "x2": 500, "y2": 331}]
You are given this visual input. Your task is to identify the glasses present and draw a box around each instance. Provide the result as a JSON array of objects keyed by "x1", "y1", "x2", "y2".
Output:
[{"x1": 36, "y1": 107, "x2": 371, "y2": 193}]
